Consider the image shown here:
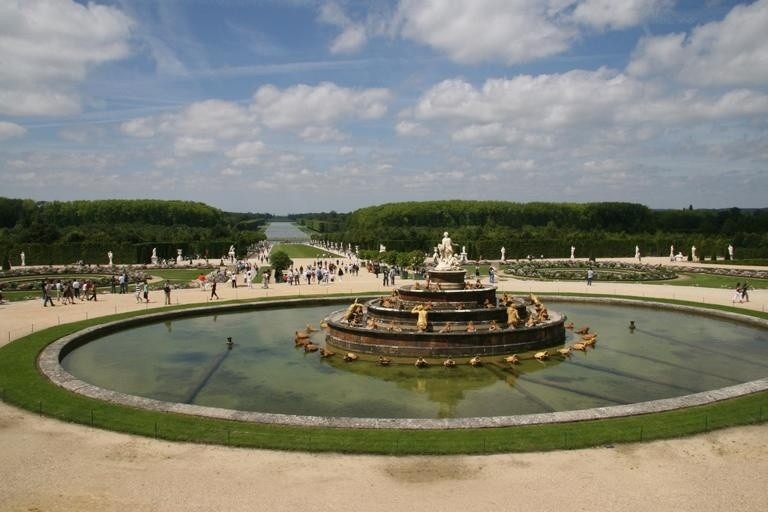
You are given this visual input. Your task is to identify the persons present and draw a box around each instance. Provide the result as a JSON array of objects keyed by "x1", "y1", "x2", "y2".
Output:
[
  {"x1": 489, "y1": 267, "x2": 494, "y2": 282},
  {"x1": 340, "y1": 278, "x2": 549, "y2": 334},
  {"x1": 587, "y1": 268, "x2": 593, "y2": 287},
  {"x1": 40, "y1": 240, "x2": 427, "y2": 307},
  {"x1": 741, "y1": 279, "x2": 750, "y2": 302},
  {"x1": 433, "y1": 231, "x2": 460, "y2": 267},
  {"x1": 475, "y1": 268, "x2": 479, "y2": 280},
  {"x1": 732, "y1": 282, "x2": 743, "y2": 303}
]
